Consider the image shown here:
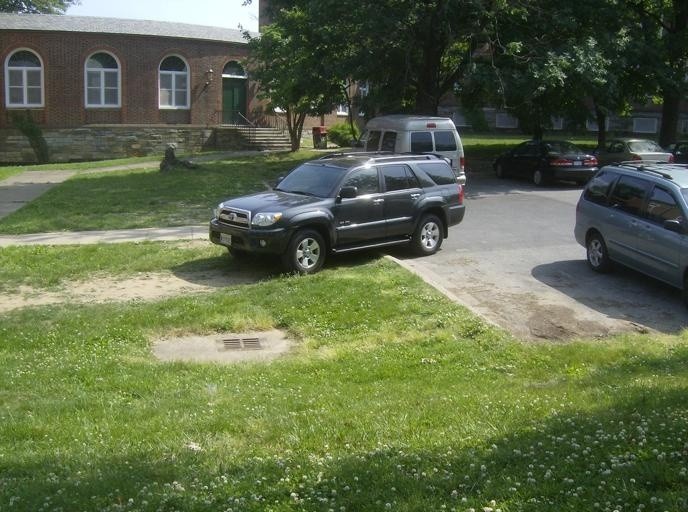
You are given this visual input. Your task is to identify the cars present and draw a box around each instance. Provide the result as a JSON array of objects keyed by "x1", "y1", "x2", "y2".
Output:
[
  {"x1": 494, "y1": 137, "x2": 688, "y2": 186},
  {"x1": 574, "y1": 160, "x2": 688, "y2": 293}
]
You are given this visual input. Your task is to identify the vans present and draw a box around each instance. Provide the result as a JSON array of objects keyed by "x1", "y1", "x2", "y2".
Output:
[{"x1": 352, "y1": 113, "x2": 464, "y2": 171}]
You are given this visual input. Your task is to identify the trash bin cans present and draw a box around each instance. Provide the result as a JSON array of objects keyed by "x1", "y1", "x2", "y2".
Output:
[{"x1": 313, "y1": 126, "x2": 328, "y2": 149}]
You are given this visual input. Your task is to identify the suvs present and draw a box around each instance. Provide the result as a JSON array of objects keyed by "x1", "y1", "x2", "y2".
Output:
[{"x1": 209, "y1": 152, "x2": 464, "y2": 276}]
